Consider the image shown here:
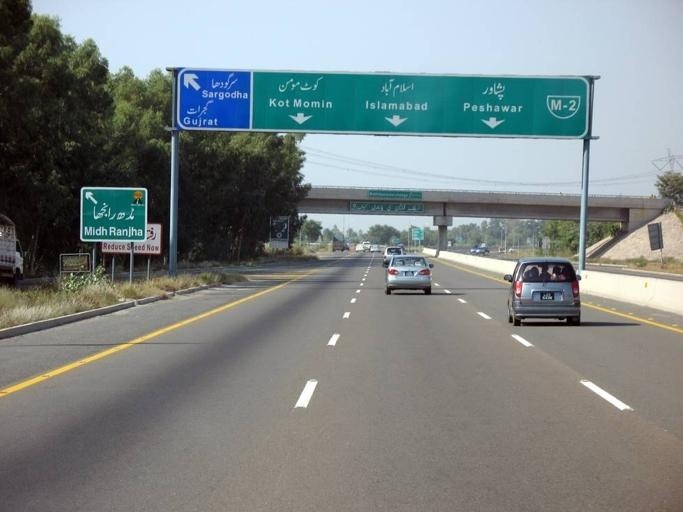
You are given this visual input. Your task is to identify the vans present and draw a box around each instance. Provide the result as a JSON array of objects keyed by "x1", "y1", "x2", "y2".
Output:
[
  {"x1": 503, "y1": 257, "x2": 583, "y2": 327},
  {"x1": 508, "y1": 246, "x2": 519, "y2": 252}
]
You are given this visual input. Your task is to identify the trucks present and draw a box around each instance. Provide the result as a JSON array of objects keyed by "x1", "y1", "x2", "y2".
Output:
[
  {"x1": 0, "y1": 213, "x2": 24, "y2": 288},
  {"x1": 390, "y1": 238, "x2": 400, "y2": 246}
]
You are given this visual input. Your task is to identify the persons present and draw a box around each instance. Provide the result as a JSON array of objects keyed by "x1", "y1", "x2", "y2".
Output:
[{"x1": 523, "y1": 265, "x2": 566, "y2": 280}]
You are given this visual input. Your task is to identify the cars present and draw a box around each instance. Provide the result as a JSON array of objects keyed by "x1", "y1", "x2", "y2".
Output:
[
  {"x1": 354, "y1": 238, "x2": 384, "y2": 254},
  {"x1": 382, "y1": 246, "x2": 405, "y2": 267},
  {"x1": 398, "y1": 244, "x2": 406, "y2": 255},
  {"x1": 470, "y1": 246, "x2": 490, "y2": 255},
  {"x1": 384, "y1": 255, "x2": 435, "y2": 295}
]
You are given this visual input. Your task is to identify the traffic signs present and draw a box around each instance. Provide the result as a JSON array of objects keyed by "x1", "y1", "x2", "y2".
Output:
[
  {"x1": 102, "y1": 224, "x2": 162, "y2": 254},
  {"x1": 268, "y1": 214, "x2": 289, "y2": 242},
  {"x1": 411, "y1": 227, "x2": 425, "y2": 240},
  {"x1": 177, "y1": 68, "x2": 592, "y2": 139},
  {"x1": 348, "y1": 201, "x2": 426, "y2": 214},
  {"x1": 367, "y1": 190, "x2": 425, "y2": 201},
  {"x1": 80, "y1": 187, "x2": 147, "y2": 241}
]
